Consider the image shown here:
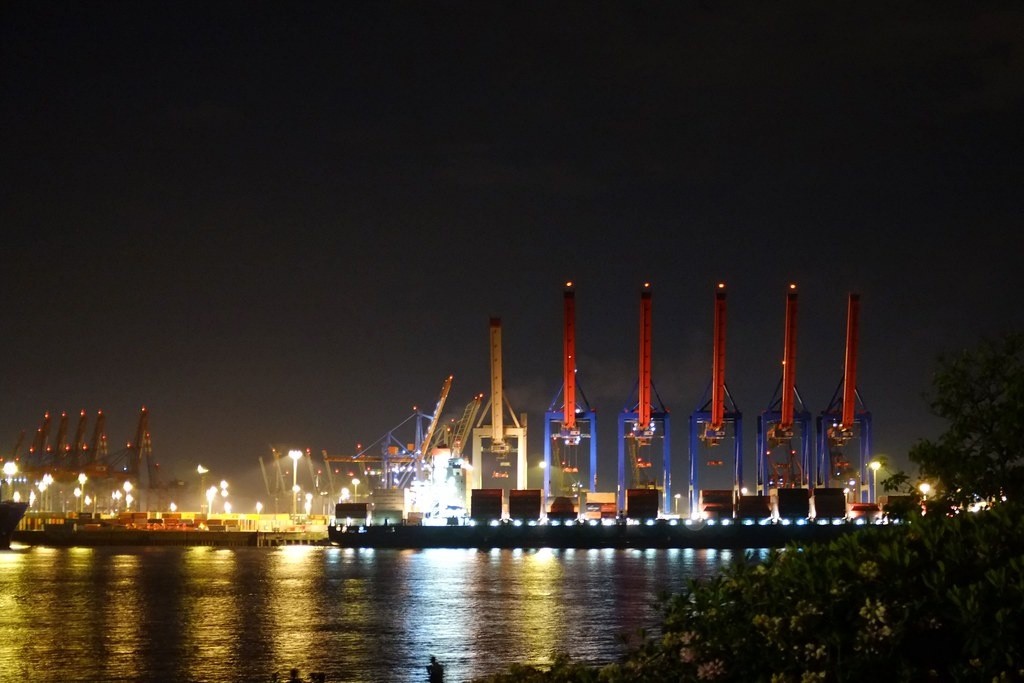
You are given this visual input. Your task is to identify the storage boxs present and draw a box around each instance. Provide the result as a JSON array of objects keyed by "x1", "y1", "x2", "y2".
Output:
[{"x1": 17, "y1": 498, "x2": 258, "y2": 532}]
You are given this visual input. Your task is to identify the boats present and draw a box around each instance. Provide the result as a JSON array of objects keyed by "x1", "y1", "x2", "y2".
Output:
[{"x1": 0, "y1": 500, "x2": 30, "y2": 551}]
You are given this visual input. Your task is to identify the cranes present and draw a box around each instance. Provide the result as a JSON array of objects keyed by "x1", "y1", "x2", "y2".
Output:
[{"x1": 1, "y1": 280, "x2": 899, "y2": 515}]
